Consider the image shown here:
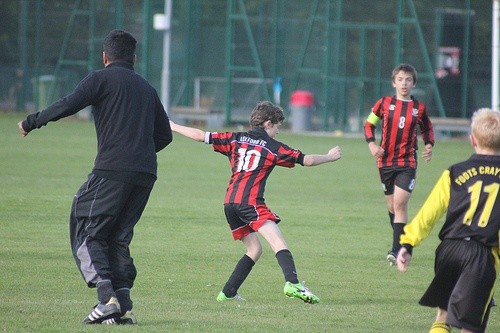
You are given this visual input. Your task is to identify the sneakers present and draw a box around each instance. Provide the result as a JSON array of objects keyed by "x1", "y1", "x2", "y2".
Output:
[
  {"x1": 387, "y1": 251, "x2": 398, "y2": 266},
  {"x1": 81, "y1": 296, "x2": 121, "y2": 324},
  {"x1": 283, "y1": 281, "x2": 320, "y2": 304},
  {"x1": 101, "y1": 310, "x2": 138, "y2": 324},
  {"x1": 217, "y1": 290, "x2": 245, "y2": 302}
]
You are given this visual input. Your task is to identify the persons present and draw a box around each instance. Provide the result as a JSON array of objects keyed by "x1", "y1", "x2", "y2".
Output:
[
  {"x1": 364, "y1": 64, "x2": 436, "y2": 263},
  {"x1": 17, "y1": 29, "x2": 173, "y2": 325},
  {"x1": 396, "y1": 107, "x2": 500, "y2": 333},
  {"x1": 168, "y1": 100, "x2": 342, "y2": 304}
]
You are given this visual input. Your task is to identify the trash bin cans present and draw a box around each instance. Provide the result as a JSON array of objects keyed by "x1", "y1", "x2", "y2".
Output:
[
  {"x1": 288, "y1": 91, "x2": 315, "y2": 132},
  {"x1": 32, "y1": 76, "x2": 64, "y2": 112}
]
